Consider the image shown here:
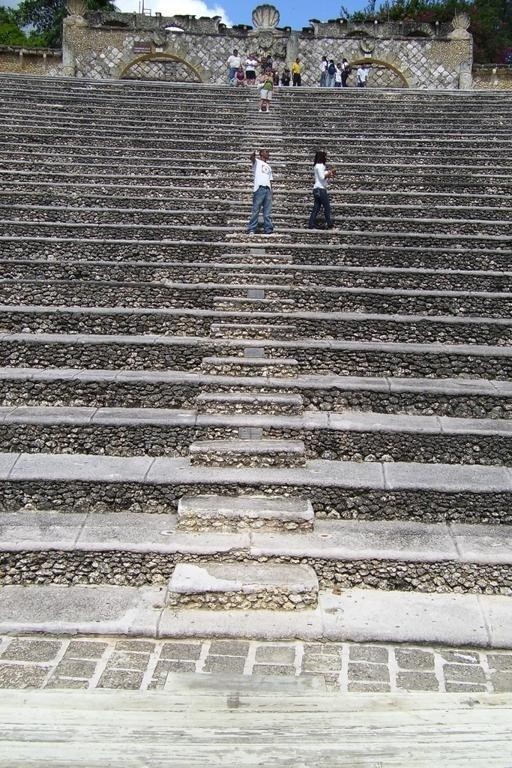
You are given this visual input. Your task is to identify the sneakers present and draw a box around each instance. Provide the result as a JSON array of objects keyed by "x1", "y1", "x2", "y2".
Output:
[
  {"x1": 266, "y1": 229, "x2": 273, "y2": 233},
  {"x1": 327, "y1": 220, "x2": 334, "y2": 228},
  {"x1": 308, "y1": 222, "x2": 321, "y2": 229},
  {"x1": 249, "y1": 231, "x2": 254, "y2": 235}
]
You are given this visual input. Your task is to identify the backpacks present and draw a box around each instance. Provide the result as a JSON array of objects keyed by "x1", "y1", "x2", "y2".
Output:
[
  {"x1": 263, "y1": 79, "x2": 273, "y2": 90},
  {"x1": 345, "y1": 64, "x2": 351, "y2": 74},
  {"x1": 328, "y1": 64, "x2": 336, "y2": 74}
]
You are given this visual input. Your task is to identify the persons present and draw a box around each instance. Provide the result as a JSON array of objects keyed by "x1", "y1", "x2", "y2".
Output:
[
  {"x1": 226, "y1": 49, "x2": 303, "y2": 87},
  {"x1": 247, "y1": 149, "x2": 274, "y2": 234},
  {"x1": 318, "y1": 56, "x2": 370, "y2": 87},
  {"x1": 256, "y1": 67, "x2": 274, "y2": 112},
  {"x1": 308, "y1": 151, "x2": 337, "y2": 229}
]
